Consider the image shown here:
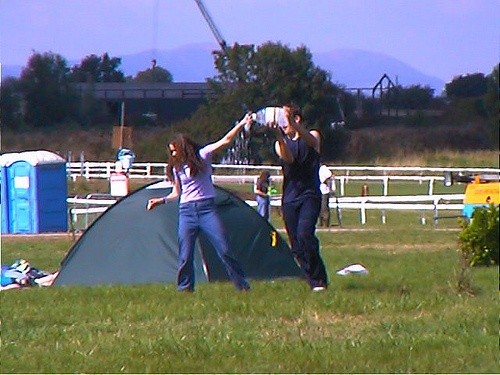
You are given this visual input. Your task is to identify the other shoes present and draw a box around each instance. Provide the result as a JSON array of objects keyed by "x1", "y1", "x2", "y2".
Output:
[{"x1": 313, "y1": 286, "x2": 325, "y2": 291}]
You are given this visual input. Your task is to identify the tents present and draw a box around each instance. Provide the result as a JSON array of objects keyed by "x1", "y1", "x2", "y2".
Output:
[{"x1": 49, "y1": 177, "x2": 304, "y2": 287}]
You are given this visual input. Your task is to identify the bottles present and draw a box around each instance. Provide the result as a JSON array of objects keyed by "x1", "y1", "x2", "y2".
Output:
[{"x1": 252, "y1": 107, "x2": 288, "y2": 128}]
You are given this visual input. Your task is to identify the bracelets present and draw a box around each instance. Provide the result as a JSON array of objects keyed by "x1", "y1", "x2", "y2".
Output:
[
  {"x1": 241, "y1": 120, "x2": 246, "y2": 126},
  {"x1": 279, "y1": 139, "x2": 285, "y2": 145},
  {"x1": 163, "y1": 196, "x2": 168, "y2": 204}
]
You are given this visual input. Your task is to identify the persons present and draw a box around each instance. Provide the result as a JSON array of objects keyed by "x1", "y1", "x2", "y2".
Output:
[
  {"x1": 144, "y1": 110, "x2": 252, "y2": 293},
  {"x1": 253, "y1": 170, "x2": 271, "y2": 222},
  {"x1": 260, "y1": 101, "x2": 328, "y2": 291},
  {"x1": 315, "y1": 164, "x2": 333, "y2": 230}
]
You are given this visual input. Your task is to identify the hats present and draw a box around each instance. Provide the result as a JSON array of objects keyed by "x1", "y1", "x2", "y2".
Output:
[{"x1": 336, "y1": 263, "x2": 369, "y2": 276}]
